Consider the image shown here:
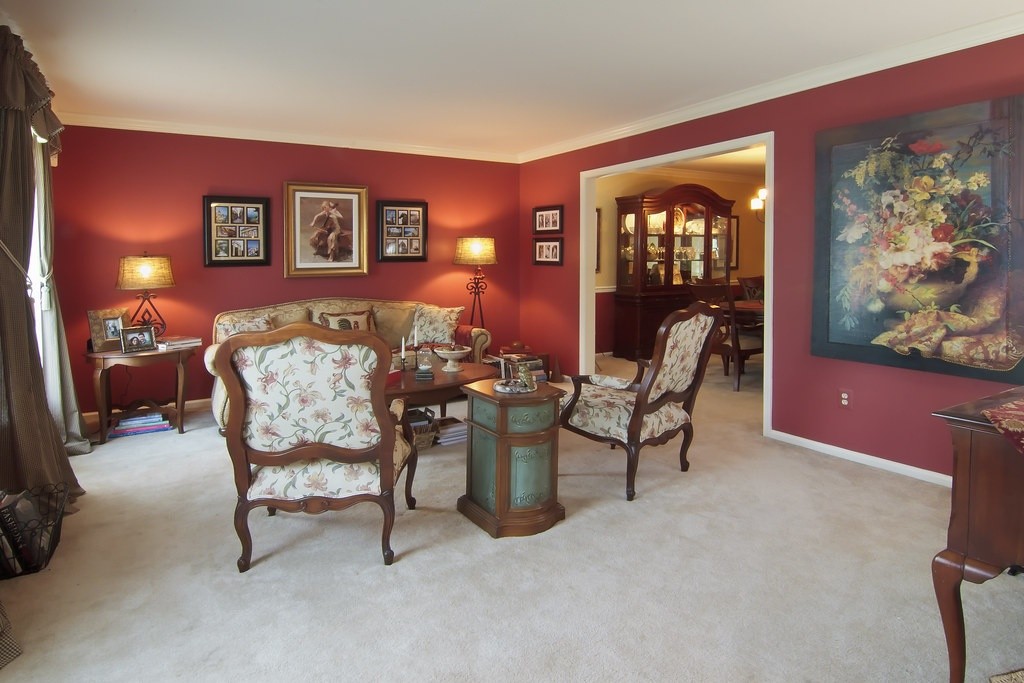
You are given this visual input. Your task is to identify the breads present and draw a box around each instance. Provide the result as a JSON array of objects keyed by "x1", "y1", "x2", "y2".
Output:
[{"x1": 441, "y1": 344, "x2": 464, "y2": 351}]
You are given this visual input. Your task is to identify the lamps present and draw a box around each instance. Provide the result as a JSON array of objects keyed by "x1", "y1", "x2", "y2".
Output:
[
  {"x1": 116, "y1": 250, "x2": 175, "y2": 337},
  {"x1": 750, "y1": 186, "x2": 767, "y2": 210},
  {"x1": 452, "y1": 235, "x2": 497, "y2": 327}
]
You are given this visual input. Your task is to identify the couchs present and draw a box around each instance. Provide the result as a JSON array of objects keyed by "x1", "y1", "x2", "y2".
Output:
[{"x1": 203, "y1": 295, "x2": 491, "y2": 438}]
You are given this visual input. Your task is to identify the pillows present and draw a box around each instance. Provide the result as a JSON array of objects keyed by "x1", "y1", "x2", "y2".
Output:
[{"x1": 214, "y1": 304, "x2": 466, "y2": 350}]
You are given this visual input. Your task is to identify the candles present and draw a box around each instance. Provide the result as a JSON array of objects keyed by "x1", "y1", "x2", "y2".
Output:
[{"x1": 401, "y1": 323, "x2": 419, "y2": 359}]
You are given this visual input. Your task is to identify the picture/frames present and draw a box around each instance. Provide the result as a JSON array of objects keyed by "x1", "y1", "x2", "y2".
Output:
[
  {"x1": 204, "y1": 182, "x2": 564, "y2": 279},
  {"x1": 87, "y1": 308, "x2": 157, "y2": 352},
  {"x1": 596, "y1": 209, "x2": 739, "y2": 273},
  {"x1": 811, "y1": 91, "x2": 1024, "y2": 385}
]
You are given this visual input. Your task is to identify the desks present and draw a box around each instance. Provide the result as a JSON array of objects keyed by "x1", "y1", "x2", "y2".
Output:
[
  {"x1": 931, "y1": 385, "x2": 1024, "y2": 683},
  {"x1": 372, "y1": 362, "x2": 500, "y2": 510},
  {"x1": 719, "y1": 299, "x2": 764, "y2": 379},
  {"x1": 82, "y1": 335, "x2": 203, "y2": 446}
]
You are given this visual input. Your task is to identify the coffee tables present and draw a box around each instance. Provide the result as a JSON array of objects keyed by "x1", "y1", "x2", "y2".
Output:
[{"x1": 457, "y1": 377, "x2": 567, "y2": 538}]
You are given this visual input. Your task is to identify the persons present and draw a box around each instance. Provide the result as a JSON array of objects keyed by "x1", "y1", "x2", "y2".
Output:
[{"x1": 130, "y1": 332, "x2": 148, "y2": 346}]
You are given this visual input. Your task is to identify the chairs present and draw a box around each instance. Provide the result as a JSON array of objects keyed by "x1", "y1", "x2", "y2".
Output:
[
  {"x1": 554, "y1": 301, "x2": 725, "y2": 501},
  {"x1": 214, "y1": 320, "x2": 418, "y2": 573},
  {"x1": 738, "y1": 275, "x2": 765, "y2": 330}
]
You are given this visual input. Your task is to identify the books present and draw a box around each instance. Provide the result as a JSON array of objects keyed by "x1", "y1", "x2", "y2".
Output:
[
  {"x1": 482, "y1": 346, "x2": 547, "y2": 382},
  {"x1": 108, "y1": 414, "x2": 173, "y2": 438},
  {"x1": 406, "y1": 409, "x2": 428, "y2": 426},
  {"x1": 432, "y1": 415, "x2": 468, "y2": 447},
  {"x1": 156, "y1": 335, "x2": 203, "y2": 349}
]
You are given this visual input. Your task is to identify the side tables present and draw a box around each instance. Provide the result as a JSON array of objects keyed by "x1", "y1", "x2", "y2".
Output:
[{"x1": 500, "y1": 346, "x2": 532, "y2": 357}]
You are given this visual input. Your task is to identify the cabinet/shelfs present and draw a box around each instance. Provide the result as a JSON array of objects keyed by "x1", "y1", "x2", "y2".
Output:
[{"x1": 613, "y1": 182, "x2": 735, "y2": 362}]
